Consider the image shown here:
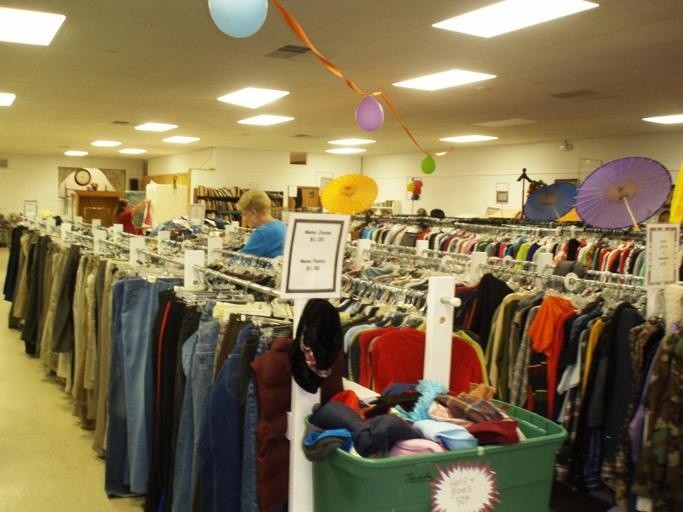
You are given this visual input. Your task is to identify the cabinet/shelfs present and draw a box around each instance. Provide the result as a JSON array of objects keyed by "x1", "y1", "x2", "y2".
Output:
[{"x1": 197, "y1": 196, "x2": 240, "y2": 215}]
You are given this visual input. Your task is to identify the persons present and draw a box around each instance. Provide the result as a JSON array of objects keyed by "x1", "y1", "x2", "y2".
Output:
[
  {"x1": 234, "y1": 186, "x2": 288, "y2": 260},
  {"x1": 112, "y1": 196, "x2": 152, "y2": 235}
]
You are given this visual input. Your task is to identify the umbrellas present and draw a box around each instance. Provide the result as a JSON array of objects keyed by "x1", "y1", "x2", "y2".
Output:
[
  {"x1": 319, "y1": 172, "x2": 380, "y2": 219},
  {"x1": 573, "y1": 154, "x2": 674, "y2": 231},
  {"x1": 523, "y1": 182, "x2": 577, "y2": 226}
]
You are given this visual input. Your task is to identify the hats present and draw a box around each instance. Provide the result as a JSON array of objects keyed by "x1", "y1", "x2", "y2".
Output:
[{"x1": 290, "y1": 297, "x2": 345, "y2": 394}]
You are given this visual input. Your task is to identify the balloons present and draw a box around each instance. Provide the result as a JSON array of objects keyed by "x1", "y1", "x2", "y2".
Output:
[
  {"x1": 420, "y1": 155, "x2": 436, "y2": 174},
  {"x1": 406, "y1": 180, "x2": 422, "y2": 200},
  {"x1": 206, "y1": 1, "x2": 270, "y2": 39},
  {"x1": 354, "y1": 95, "x2": 383, "y2": 131}
]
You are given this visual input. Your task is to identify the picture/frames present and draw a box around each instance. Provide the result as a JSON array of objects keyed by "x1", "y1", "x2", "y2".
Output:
[{"x1": 23, "y1": 200, "x2": 37, "y2": 216}]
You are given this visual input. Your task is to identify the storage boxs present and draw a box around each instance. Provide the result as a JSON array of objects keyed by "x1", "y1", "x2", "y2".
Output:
[
  {"x1": 304, "y1": 399, "x2": 568, "y2": 512},
  {"x1": 297, "y1": 187, "x2": 321, "y2": 207}
]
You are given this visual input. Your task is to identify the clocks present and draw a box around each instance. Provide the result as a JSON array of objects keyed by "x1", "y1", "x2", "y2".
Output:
[{"x1": 75, "y1": 169, "x2": 91, "y2": 185}]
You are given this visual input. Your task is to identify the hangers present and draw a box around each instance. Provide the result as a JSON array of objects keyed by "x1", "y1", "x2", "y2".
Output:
[
  {"x1": 340, "y1": 210, "x2": 683, "y2": 335},
  {"x1": 19, "y1": 216, "x2": 293, "y2": 339}
]
